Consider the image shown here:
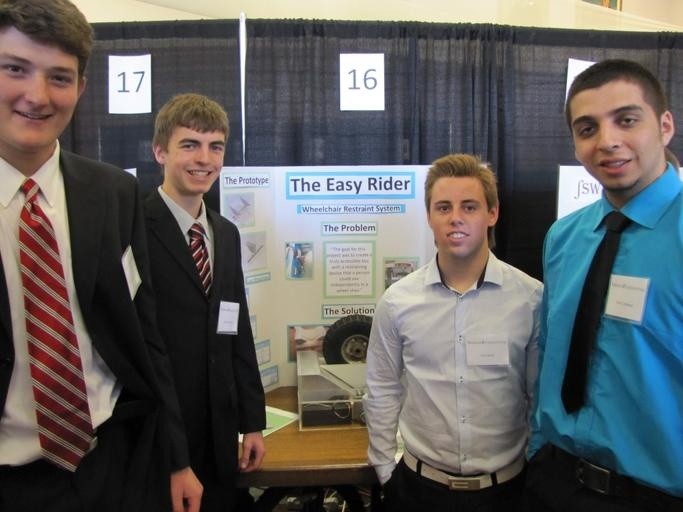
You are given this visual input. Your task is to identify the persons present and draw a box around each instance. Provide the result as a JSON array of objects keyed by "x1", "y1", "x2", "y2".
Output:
[
  {"x1": 361, "y1": 152, "x2": 548, "y2": 511},
  {"x1": 521, "y1": 59, "x2": 683, "y2": 509},
  {"x1": 0, "y1": 2, "x2": 211, "y2": 511},
  {"x1": 142, "y1": 89, "x2": 269, "y2": 511}
]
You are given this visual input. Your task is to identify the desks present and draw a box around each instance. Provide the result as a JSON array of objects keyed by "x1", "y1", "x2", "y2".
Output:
[{"x1": 231, "y1": 385, "x2": 377, "y2": 508}]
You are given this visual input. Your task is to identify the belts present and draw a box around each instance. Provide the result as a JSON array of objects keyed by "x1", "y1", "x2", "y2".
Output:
[
  {"x1": 402, "y1": 448, "x2": 527, "y2": 491},
  {"x1": 551, "y1": 445, "x2": 680, "y2": 511}
]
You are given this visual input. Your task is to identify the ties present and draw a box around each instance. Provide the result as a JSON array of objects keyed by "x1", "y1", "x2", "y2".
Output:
[
  {"x1": 16, "y1": 180, "x2": 95, "y2": 481},
  {"x1": 560, "y1": 211, "x2": 634, "y2": 415},
  {"x1": 188, "y1": 224, "x2": 213, "y2": 296}
]
